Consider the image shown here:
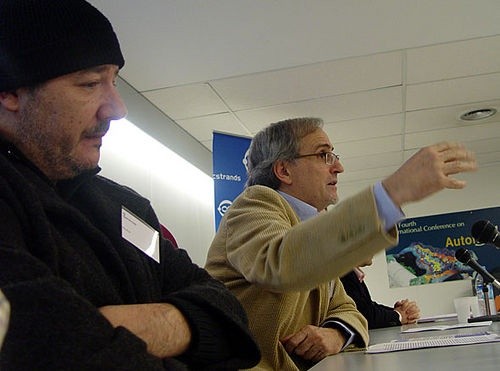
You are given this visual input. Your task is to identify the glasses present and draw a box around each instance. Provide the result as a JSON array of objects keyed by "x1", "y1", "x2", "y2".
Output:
[{"x1": 294, "y1": 152, "x2": 340, "y2": 166}]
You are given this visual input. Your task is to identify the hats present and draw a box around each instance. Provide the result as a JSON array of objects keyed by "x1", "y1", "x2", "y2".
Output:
[{"x1": 0, "y1": 0, "x2": 125, "y2": 97}]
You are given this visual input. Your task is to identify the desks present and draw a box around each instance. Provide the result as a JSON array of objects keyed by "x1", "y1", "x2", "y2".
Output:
[{"x1": 307, "y1": 317, "x2": 500, "y2": 371}]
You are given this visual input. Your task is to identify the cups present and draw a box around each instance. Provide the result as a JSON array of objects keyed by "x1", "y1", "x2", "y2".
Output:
[
  {"x1": 469, "y1": 296, "x2": 482, "y2": 318},
  {"x1": 454, "y1": 297, "x2": 471, "y2": 323}
]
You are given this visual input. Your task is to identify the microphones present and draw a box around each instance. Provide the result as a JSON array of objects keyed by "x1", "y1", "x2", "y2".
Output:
[
  {"x1": 455, "y1": 246, "x2": 500, "y2": 291},
  {"x1": 471, "y1": 219, "x2": 500, "y2": 248}
]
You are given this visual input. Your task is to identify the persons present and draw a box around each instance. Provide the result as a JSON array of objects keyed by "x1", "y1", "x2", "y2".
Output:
[
  {"x1": 339, "y1": 256, "x2": 421, "y2": 330},
  {"x1": 203, "y1": 117, "x2": 477, "y2": 371},
  {"x1": 0, "y1": 0, "x2": 262, "y2": 371}
]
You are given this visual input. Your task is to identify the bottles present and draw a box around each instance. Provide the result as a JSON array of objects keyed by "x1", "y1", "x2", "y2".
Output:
[{"x1": 475, "y1": 266, "x2": 496, "y2": 316}]
